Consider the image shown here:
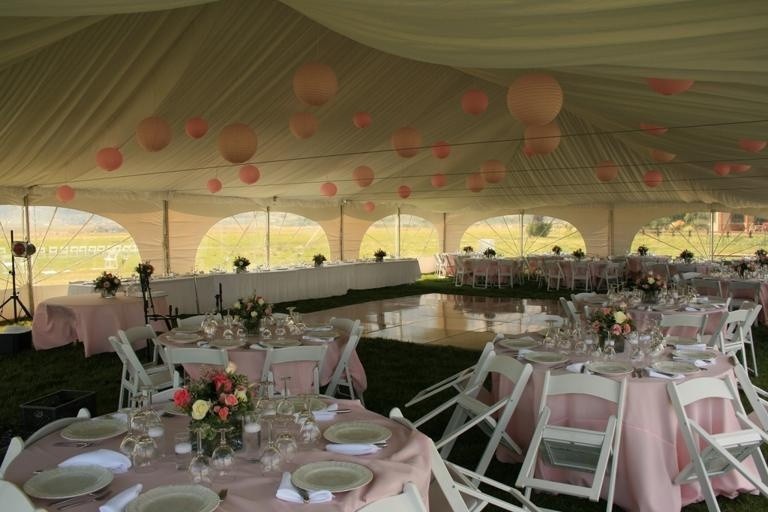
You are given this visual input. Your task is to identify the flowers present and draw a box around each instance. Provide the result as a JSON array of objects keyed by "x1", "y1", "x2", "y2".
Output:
[
  {"x1": 679, "y1": 249, "x2": 693, "y2": 260},
  {"x1": 552, "y1": 246, "x2": 561, "y2": 255},
  {"x1": 374, "y1": 248, "x2": 386, "y2": 258},
  {"x1": 464, "y1": 246, "x2": 474, "y2": 254},
  {"x1": 638, "y1": 245, "x2": 648, "y2": 254},
  {"x1": 483, "y1": 247, "x2": 496, "y2": 257},
  {"x1": 754, "y1": 249, "x2": 768, "y2": 261},
  {"x1": 572, "y1": 247, "x2": 584, "y2": 259}
]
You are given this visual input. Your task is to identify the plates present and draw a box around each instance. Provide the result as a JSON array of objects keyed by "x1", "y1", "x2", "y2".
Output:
[
  {"x1": 21, "y1": 464, "x2": 115, "y2": 499},
  {"x1": 323, "y1": 421, "x2": 392, "y2": 445},
  {"x1": 290, "y1": 461, "x2": 373, "y2": 494},
  {"x1": 61, "y1": 419, "x2": 127, "y2": 441},
  {"x1": 125, "y1": 484, "x2": 221, "y2": 512}
]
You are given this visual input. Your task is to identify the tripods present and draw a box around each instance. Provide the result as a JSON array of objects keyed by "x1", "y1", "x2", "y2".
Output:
[{"x1": 0, "y1": 230, "x2": 33, "y2": 324}]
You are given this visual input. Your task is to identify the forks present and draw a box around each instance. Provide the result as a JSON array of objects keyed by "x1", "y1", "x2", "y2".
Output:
[{"x1": 213, "y1": 488, "x2": 227, "y2": 511}]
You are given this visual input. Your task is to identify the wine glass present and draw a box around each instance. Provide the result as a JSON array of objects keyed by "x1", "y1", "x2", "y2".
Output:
[
  {"x1": 122, "y1": 379, "x2": 327, "y2": 486},
  {"x1": 450, "y1": 251, "x2": 767, "y2": 378},
  {"x1": 168, "y1": 304, "x2": 339, "y2": 344}
]
